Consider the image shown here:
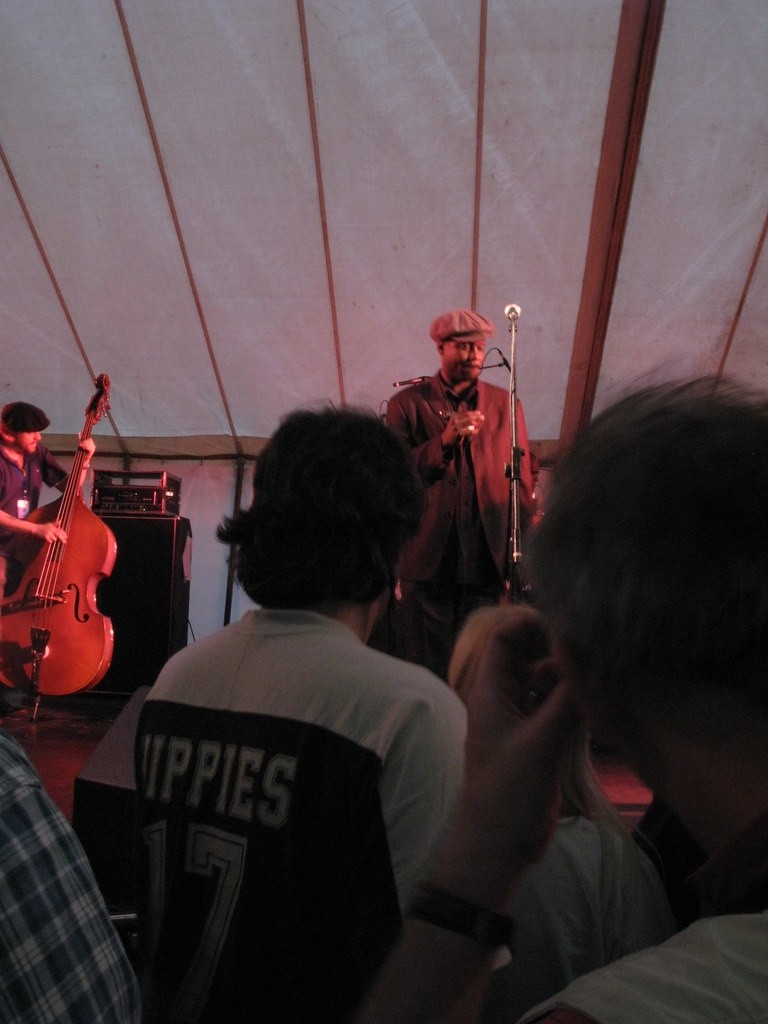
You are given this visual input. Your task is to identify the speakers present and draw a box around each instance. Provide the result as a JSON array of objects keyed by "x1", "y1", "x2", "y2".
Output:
[{"x1": 84, "y1": 513, "x2": 192, "y2": 696}]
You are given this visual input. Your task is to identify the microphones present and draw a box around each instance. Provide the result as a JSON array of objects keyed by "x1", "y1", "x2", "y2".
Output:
[
  {"x1": 504, "y1": 305, "x2": 521, "y2": 320},
  {"x1": 497, "y1": 350, "x2": 512, "y2": 374}
]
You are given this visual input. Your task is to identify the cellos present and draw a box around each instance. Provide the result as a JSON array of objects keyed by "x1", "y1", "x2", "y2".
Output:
[{"x1": 0, "y1": 370, "x2": 122, "y2": 739}]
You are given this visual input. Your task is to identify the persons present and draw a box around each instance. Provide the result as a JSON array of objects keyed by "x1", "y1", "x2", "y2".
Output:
[
  {"x1": 0, "y1": 375, "x2": 768, "y2": 1024},
  {"x1": 386, "y1": 307, "x2": 536, "y2": 635}
]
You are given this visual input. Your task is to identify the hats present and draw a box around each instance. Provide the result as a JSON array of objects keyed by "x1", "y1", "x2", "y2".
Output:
[
  {"x1": 430, "y1": 307, "x2": 498, "y2": 342},
  {"x1": 0, "y1": 401, "x2": 54, "y2": 434}
]
